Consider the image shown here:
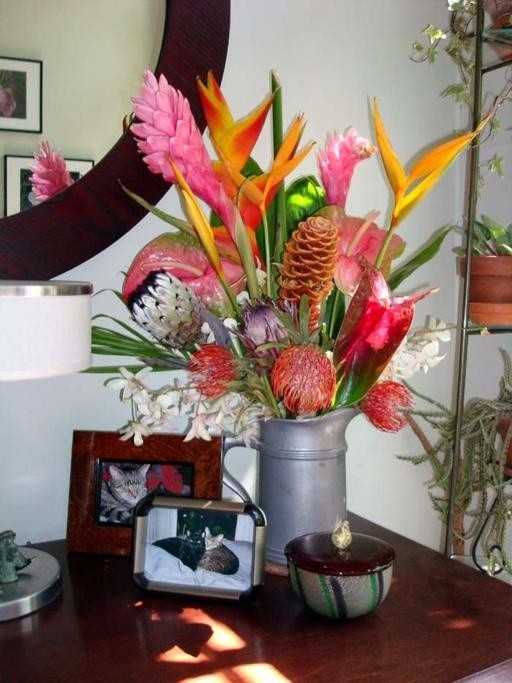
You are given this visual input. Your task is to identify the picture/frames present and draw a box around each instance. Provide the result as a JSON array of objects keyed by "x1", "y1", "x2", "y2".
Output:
[
  {"x1": 0, "y1": 55, "x2": 47, "y2": 134},
  {"x1": 65, "y1": 428, "x2": 267, "y2": 601},
  {"x1": 8, "y1": 155, "x2": 94, "y2": 217}
]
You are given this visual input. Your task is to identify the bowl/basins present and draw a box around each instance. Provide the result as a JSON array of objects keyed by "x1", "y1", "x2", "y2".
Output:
[{"x1": 284, "y1": 522, "x2": 397, "y2": 619}]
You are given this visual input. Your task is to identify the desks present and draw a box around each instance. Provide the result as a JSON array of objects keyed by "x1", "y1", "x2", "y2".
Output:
[{"x1": 3, "y1": 513, "x2": 509, "y2": 680}]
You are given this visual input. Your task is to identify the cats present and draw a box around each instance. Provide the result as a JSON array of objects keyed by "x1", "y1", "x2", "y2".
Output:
[
  {"x1": 151, "y1": 529, "x2": 206, "y2": 572},
  {"x1": 100, "y1": 463, "x2": 151, "y2": 523},
  {"x1": 198, "y1": 525, "x2": 239, "y2": 575}
]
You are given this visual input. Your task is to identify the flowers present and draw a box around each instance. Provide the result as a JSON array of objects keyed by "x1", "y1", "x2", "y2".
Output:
[
  {"x1": 29, "y1": 141, "x2": 75, "y2": 203},
  {"x1": 88, "y1": 71, "x2": 511, "y2": 445}
]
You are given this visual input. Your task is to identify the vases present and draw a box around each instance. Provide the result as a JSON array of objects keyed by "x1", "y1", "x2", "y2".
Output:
[{"x1": 250, "y1": 404, "x2": 371, "y2": 574}]
